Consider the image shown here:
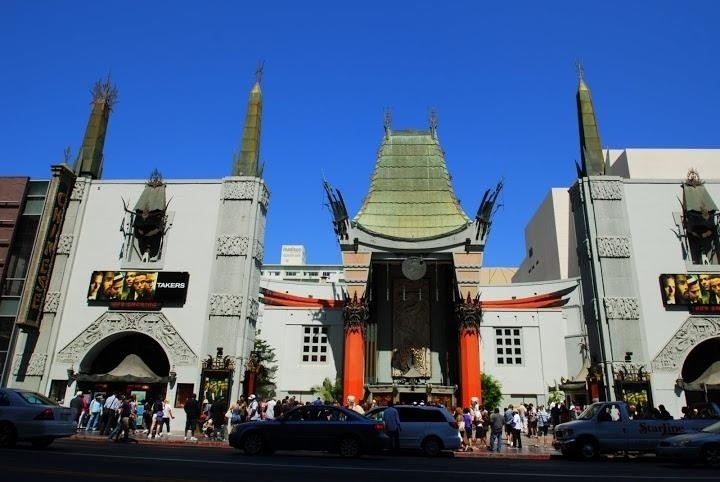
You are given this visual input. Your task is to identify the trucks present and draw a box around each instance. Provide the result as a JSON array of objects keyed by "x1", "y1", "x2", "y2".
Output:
[{"x1": 553, "y1": 402, "x2": 719, "y2": 459}]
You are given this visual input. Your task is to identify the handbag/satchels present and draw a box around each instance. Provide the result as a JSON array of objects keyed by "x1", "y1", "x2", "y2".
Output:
[{"x1": 102, "y1": 414, "x2": 107, "y2": 421}]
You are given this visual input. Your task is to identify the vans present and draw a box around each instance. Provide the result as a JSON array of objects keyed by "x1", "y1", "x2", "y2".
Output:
[{"x1": 362, "y1": 403, "x2": 463, "y2": 455}]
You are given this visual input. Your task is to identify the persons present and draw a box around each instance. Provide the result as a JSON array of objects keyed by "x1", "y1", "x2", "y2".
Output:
[
  {"x1": 184, "y1": 393, "x2": 377, "y2": 442},
  {"x1": 629, "y1": 400, "x2": 711, "y2": 419},
  {"x1": 660, "y1": 274, "x2": 720, "y2": 305},
  {"x1": 88, "y1": 271, "x2": 159, "y2": 303},
  {"x1": 72, "y1": 389, "x2": 175, "y2": 442},
  {"x1": 455, "y1": 399, "x2": 587, "y2": 452},
  {"x1": 382, "y1": 400, "x2": 402, "y2": 459},
  {"x1": 598, "y1": 407, "x2": 612, "y2": 422}
]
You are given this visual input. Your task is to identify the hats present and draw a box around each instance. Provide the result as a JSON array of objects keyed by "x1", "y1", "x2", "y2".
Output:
[
  {"x1": 203, "y1": 399, "x2": 209, "y2": 404},
  {"x1": 95, "y1": 393, "x2": 103, "y2": 398},
  {"x1": 248, "y1": 394, "x2": 256, "y2": 400}
]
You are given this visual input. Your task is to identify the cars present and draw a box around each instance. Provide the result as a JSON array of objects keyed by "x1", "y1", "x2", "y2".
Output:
[
  {"x1": 1, "y1": 389, "x2": 77, "y2": 448},
  {"x1": 657, "y1": 422, "x2": 719, "y2": 465},
  {"x1": 229, "y1": 404, "x2": 390, "y2": 458}
]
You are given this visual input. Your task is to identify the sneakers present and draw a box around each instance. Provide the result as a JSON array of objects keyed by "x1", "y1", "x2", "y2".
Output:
[
  {"x1": 463, "y1": 433, "x2": 548, "y2": 452},
  {"x1": 77, "y1": 426, "x2": 198, "y2": 441}
]
